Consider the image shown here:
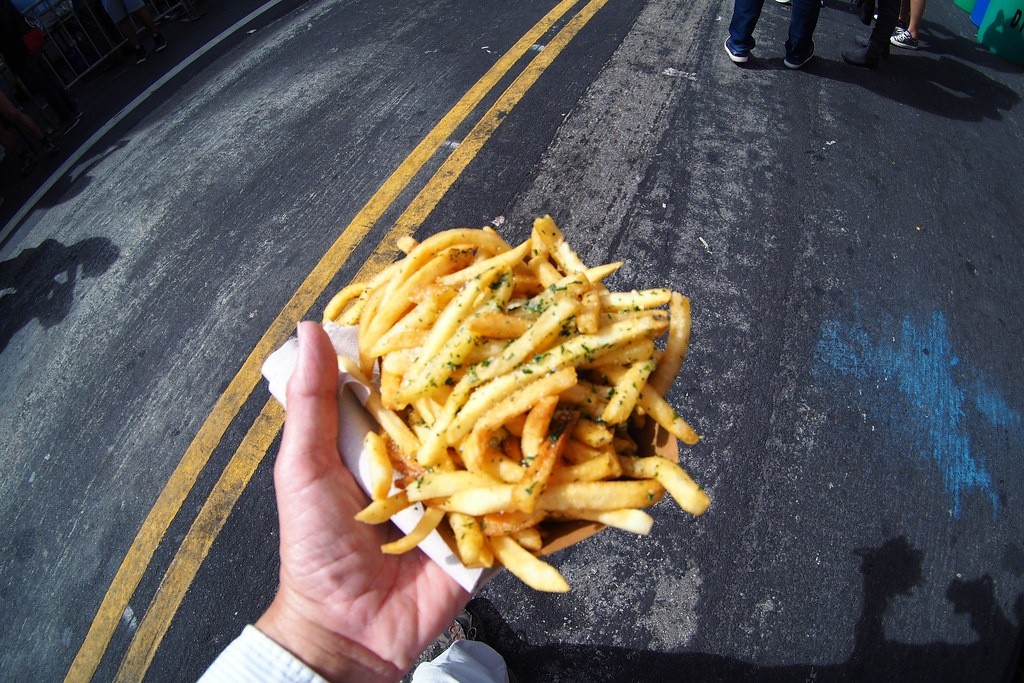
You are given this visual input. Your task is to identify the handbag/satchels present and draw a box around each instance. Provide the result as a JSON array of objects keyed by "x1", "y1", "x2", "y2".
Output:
[{"x1": 23, "y1": 28, "x2": 47, "y2": 55}]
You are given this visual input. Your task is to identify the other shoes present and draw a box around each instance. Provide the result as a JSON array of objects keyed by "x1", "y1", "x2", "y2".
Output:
[
  {"x1": 46, "y1": 109, "x2": 84, "y2": 141},
  {"x1": 842, "y1": 48, "x2": 888, "y2": 71},
  {"x1": 855, "y1": 36, "x2": 869, "y2": 47}
]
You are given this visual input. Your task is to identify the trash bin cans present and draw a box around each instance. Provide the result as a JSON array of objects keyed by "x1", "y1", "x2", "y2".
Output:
[{"x1": 954, "y1": 0, "x2": 1024, "y2": 63}]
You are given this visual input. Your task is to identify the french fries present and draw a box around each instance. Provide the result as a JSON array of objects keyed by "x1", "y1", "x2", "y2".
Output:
[{"x1": 321, "y1": 213, "x2": 709, "y2": 593}]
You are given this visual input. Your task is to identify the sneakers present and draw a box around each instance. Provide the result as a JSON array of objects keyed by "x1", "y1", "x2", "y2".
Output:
[
  {"x1": 18, "y1": 150, "x2": 37, "y2": 176},
  {"x1": 152, "y1": 31, "x2": 168, "y2": 53},
  {"x1": 784, "y1": 39, "x2": 815, "y2": 68},
  {"x1": 873, "y1": 13, "x2": 908, "y2": 32},
  {"x1": 135, "y1": 44, "x2": 147, "y2": 65},
  {"x1": 890, "y1": 30, "x2": 919, "y2": 49},
  {"x1": 38, "y1": 135, "x2": 61, "y2": 159},
  {"x1": 438, "y1": 608, "x2": 518, "y2": 683},
  {"x1": 724, "y1": 35, "x2": 748, "y2": 63}
]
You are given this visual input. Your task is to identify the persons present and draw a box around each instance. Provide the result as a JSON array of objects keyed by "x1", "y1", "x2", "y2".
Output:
[
  {"x1": 842, "y1": 0, "x2": 926, "y2": 66},
  {"x1": 725, "y1": 0, "x2": 820, "y2": 68},
  {"x1": 195, "y1": 320, "x2": 526, "y2": 683}
]
